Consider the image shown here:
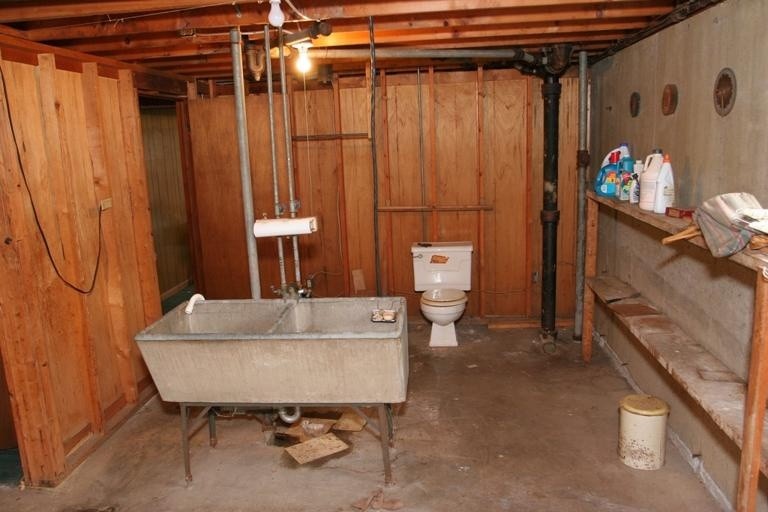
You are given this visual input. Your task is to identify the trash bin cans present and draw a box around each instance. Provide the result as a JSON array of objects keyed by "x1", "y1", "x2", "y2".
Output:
[{"x1": 617, "y1": 395, "x2": 671, "y2": 470}]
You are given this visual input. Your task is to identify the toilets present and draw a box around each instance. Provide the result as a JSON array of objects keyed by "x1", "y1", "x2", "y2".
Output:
[{"x1": 410, "y1": 241, "x2": 473, "y2": 347}]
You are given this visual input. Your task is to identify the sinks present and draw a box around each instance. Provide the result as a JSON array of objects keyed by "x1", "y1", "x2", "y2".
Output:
[
  {"x1": 134, "y1": 299, "x2": 285, "y2": 340},
  {"x1": 271, "y1": 297, "x2": 406, "y2": 339}
]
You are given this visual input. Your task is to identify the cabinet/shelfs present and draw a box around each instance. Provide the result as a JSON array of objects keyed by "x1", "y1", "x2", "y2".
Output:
[{"x1": 578, "y1": 188, "x2": 767, "y2": 511}]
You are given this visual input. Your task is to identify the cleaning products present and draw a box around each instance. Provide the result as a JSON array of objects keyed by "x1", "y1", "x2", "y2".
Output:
[{"x1": 595, "y1": 143, "x2": 675, "y2": 213}]
